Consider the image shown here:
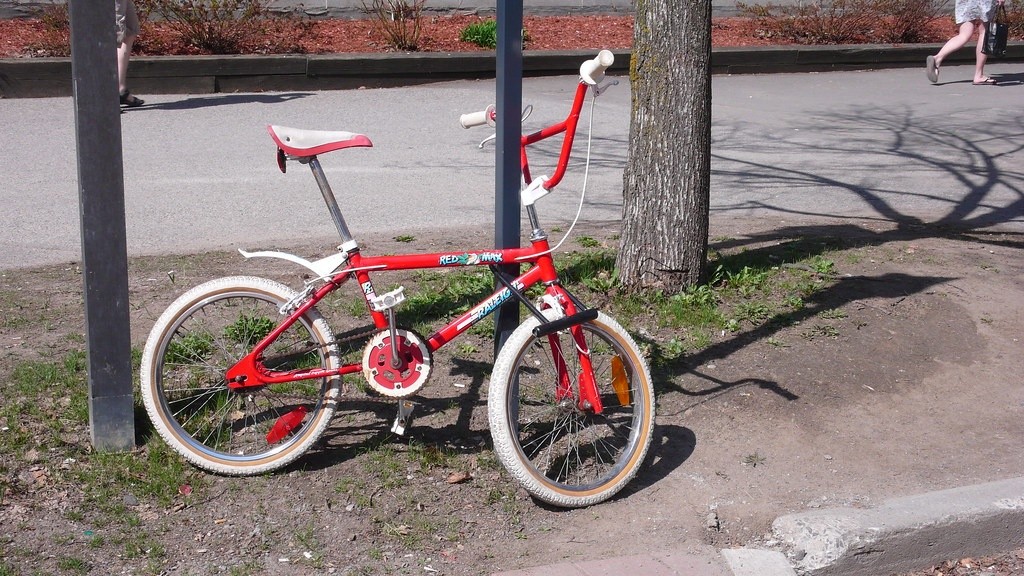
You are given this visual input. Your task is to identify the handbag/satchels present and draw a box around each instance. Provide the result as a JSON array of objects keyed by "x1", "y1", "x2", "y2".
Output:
[{"x1": 981, "y1": 4, "x2": 1008, "y2": 58}]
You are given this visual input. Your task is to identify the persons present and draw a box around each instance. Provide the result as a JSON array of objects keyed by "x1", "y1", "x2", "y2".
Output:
[{"x1": 927, "y1": 0, "x2": 1006, "y2": 85}]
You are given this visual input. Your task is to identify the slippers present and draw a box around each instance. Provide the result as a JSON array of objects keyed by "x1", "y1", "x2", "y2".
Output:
[
  {"x1": 972, "y1": 76, "x2": 998, "y2": 85},
  {"x1": 926, "y1": 55, "x2": 940, "y2": 83}
]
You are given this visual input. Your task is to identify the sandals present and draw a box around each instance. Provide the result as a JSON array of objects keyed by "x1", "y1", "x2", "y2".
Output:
[
  {"x1": 119, "y1": 89, "x2": 145, "y2": 106},
  {"x1": 120, "y1": 107, "x2": 124, "y2": 113}
]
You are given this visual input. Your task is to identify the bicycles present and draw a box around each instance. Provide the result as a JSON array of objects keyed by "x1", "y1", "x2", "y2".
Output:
[{"x1": 140, "y1": 49, "x2": 656, "y2": 508}]
